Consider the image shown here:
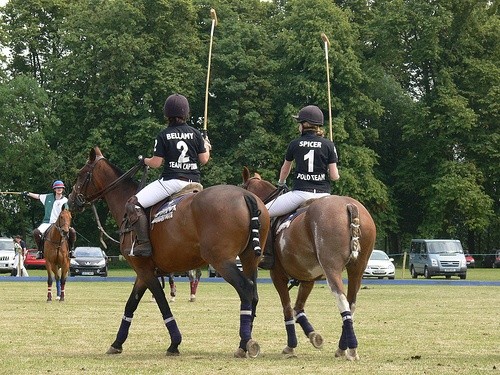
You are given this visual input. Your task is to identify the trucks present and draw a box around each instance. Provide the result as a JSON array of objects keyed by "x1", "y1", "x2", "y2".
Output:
[{"x1": 408, "y1": 238, "x2": 468, "y2": 280}]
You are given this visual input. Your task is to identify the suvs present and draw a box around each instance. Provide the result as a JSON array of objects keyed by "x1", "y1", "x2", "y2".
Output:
[
  {"x1": 482, "y1": 249, "x2": 500, "y2": 269},
  {"x1": 0, "y1": 238, "x2": 18, "y2": 276}
]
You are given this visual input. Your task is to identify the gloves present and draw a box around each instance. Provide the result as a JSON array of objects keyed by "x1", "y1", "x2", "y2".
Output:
[
  {"x1": 202, "y1": 135, "x2": 211, "y2": 150},
  {"x1": 23, "y1": 191, "x2": 29, "y2": 196},
  {"x1": 138, "y1": 156, "x2": 151, "y2": 166},
  {"x1": 278, "y1": 179, "x2": 286, "y2": 186}
]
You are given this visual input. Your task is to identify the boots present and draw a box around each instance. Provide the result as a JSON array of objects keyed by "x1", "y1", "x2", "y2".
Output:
[
  {"x1": 20, "y1": 268, "x2": 23, "y2": 276},
  {"x1": 124, "y1": 207, "x2": 152, "y2": 256},
  {"x1": 37, "y1": 242, "x2": 44, "y2": 259},
  {"x1": 68, "y1": 240, "x2": 76, "y2": 258},
  {"x1": 257, "y1": 224, "x2": 273, "y2": 269},
  {"x1": 10, "y1": 268, "x2": 16, "y2": 276}
]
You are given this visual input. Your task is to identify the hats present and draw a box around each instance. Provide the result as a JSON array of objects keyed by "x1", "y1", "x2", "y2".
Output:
[{"x1": 13, "y1": 235, "x2": 22, "y2": 238}]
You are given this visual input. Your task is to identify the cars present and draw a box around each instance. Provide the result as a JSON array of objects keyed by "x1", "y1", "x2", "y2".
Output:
[
  {"x1": 67, "y1": 247, "x2": 108, "y2": 278},
  {"x1": 464, "y1": 250, "x2": 475, "y2": 268},
  {"x1": 23, "y1": 249, "x2": 47, "y2": 268},
  {"x1": 208, "y1": 255, "x2": 243, "y2": 277},
  {"x1": 362, "y1": 250, "x2": 396, "y2": 280}
]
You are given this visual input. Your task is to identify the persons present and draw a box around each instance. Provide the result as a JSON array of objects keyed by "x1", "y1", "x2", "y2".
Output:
[
  {"x1": 125, "y1": 94, "x2": 210, "y2": 257},
  {"x1": 10, "y1": 235, "x2": 29, "y2": 277},
  {"x1": 258, "y1": 105, "x2": 340, "y2": 270},
  {"x1": 20, "y1": 181, "x2": 77, "y2": 259}
]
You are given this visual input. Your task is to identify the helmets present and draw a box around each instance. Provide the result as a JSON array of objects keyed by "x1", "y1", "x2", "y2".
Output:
[
  {"x1": 292, "y1": 105, "x2": 324, "y2": 127},
  {"x1": 164, "y1": 94, "x2": 189, "y2": 117},
  {"x1": 53, "y1": 181, "x2": 65, "y2": 189}
]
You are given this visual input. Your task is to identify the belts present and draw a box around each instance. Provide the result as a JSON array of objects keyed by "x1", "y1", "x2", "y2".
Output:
[
  {"x1": 294, "y1": 188, "x2": 325, "y2": 193},
  {"x1": 177, "y1": 178, "x2": 198, "y2": 183}
]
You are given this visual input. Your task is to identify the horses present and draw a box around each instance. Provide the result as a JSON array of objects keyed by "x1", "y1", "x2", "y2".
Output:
[
  {"x1": 241, "y1": 165, "x2": 377, "y2": 362},
  {"x1": 66, "y1": 145, "x2": 270, "y2": 360},
  {"x1": 151, "y1": 266, "x2": 202, "y2": 303},
  {"x1": 43, "y1": 204, "x2": 73, "y2": 301}
]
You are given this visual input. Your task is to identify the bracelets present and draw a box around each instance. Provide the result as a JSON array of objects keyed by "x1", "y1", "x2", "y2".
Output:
[{"x1": 278, "y1": 179, "x2": 286, "y2": 186}]
